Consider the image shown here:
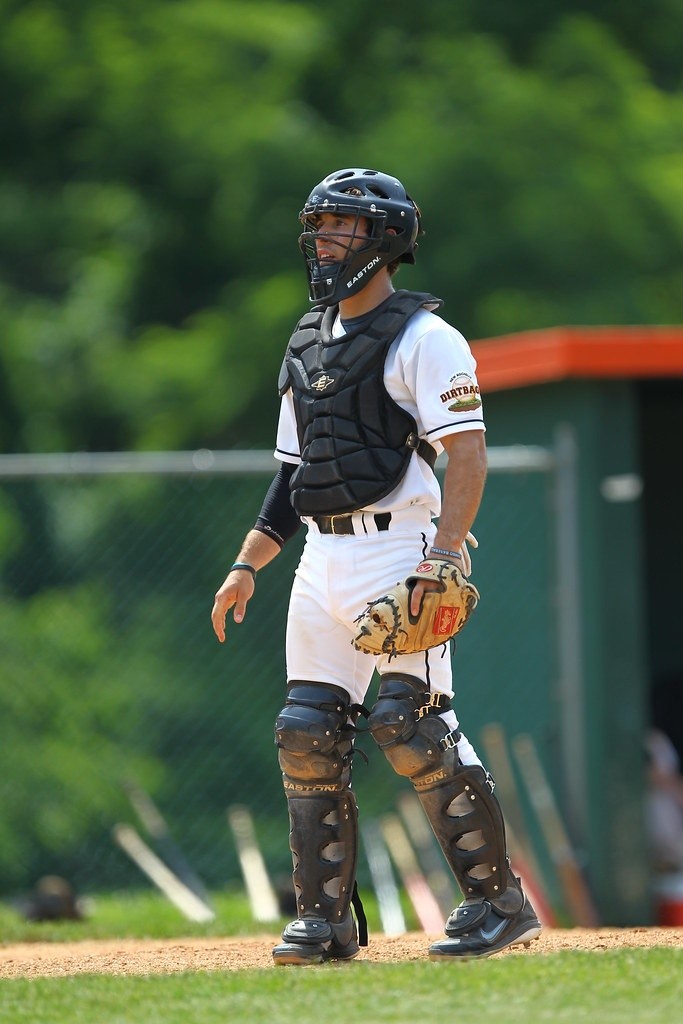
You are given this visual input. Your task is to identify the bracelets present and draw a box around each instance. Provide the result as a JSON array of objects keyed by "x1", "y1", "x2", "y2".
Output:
[
  {"x1": 430, "y1": 547, "x2": 462, "y2": 559},
  {"x1": 230, "y1": 564, "x2": 257, "y2": 578}
]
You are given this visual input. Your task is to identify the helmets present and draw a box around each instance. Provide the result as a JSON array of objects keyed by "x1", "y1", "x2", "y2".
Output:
[{"x1": 297, "y1": 168, "x2": 425, "y2": 305}]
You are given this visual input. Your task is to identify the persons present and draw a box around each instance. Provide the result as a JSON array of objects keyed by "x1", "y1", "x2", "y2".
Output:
[{"x1": 210, "y1": 167, "x2": 543, "y2": 966}]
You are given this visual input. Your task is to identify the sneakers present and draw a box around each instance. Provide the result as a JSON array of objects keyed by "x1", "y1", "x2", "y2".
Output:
[
  {"x1": 272, "y1": 919, "x2": 361, "y2": 964},
  {"x1": 429, "y1": 877, "x2": 543, "y2": 960}
]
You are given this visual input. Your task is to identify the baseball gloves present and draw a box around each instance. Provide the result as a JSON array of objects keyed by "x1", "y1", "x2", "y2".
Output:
[{"x1": 348, "y1": 557, "x2": 482, "y2": 659}]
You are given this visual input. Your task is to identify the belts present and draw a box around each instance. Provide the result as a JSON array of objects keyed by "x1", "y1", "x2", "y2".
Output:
[{"x1": 313, "y1": 512, "x2": 391, "y2": 537}]
